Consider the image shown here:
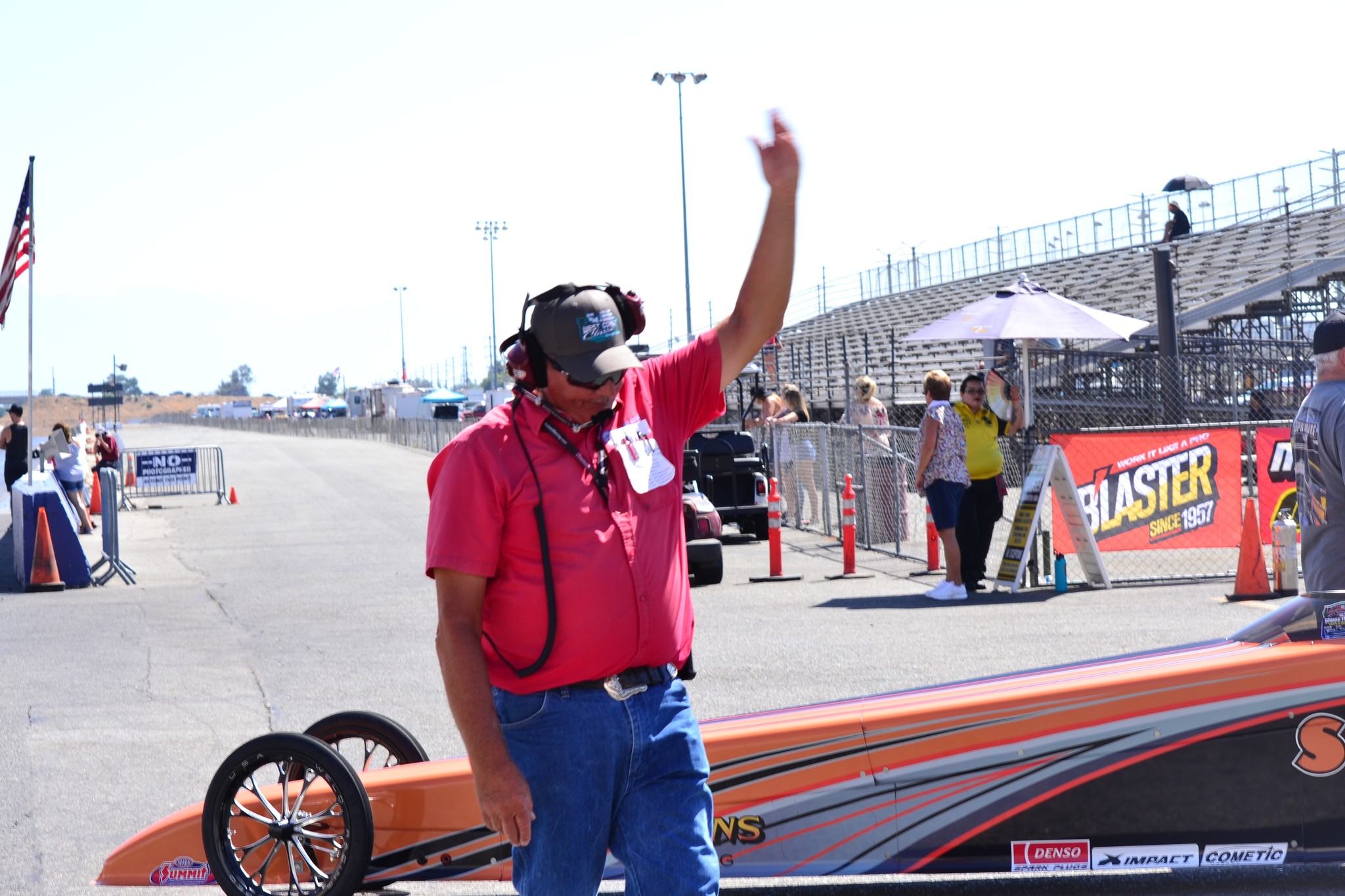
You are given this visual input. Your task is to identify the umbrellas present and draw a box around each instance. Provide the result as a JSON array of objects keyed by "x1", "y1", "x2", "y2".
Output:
[
  {"x1": 1162, "y1": 175, "x2": 1215, "y2": 232},
  {"x1": 422, "y1": 387, "x2": 469, "y2": 407},
  {"x1": 898, "y1": 272, "x2": 1151, "y2": 483},
  {"x1": 301, "y1": 397, "x2": 346, "y2": 411}
]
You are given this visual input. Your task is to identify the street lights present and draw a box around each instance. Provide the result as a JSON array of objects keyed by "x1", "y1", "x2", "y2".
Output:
[
  {"x1": 649, "y1": 72, "x2": 709, "y2": 348},
  {"x1": 392, "y1": 284, "x2": 409, "y2": 384},
  {"x1": 476, "y1": 220, "x2": 509, "y2": 391}
]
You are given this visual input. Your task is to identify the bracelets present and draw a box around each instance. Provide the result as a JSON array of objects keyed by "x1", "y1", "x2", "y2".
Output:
[
  {"x1": 755, "y1": 421, "x2": 756, "y2": 426},
  {"x1": 1012, "y1": 397, "x2": 1019, "y2": 401}
]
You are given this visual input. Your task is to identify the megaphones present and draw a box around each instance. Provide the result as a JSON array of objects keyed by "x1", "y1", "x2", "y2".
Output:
[{"x1": 39, "y1": 428, "x2": 72, "y2": 461}]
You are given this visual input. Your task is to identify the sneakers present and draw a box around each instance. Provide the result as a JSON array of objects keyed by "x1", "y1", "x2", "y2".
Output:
[
  {"x1": 931, "y1": 581, "x2": 968, "y2": 600},
  {"x1": 925, "y1": 580, "x2": 951, "y2": 597}
]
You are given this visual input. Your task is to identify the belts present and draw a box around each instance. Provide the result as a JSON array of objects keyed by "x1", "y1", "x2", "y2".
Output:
[{"x1": 574, "y1": 661, "x2": 679, "y2": 702}]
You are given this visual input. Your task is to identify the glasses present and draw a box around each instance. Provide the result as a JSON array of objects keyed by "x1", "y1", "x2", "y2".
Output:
[
  {"x1": 965, "y1": 388, "x2": 985, "y2": 395},
  {"x1": 543, "y1": 354, "x2": 628, "y2": 390},
  {"x1": 922, "y1": 388, "x2": 925, "y2": 394}
]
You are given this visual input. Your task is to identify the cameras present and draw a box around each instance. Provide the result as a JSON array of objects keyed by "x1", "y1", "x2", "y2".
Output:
[{"x1": 95, "y1": 434, "x2": 101, "y2": 437}]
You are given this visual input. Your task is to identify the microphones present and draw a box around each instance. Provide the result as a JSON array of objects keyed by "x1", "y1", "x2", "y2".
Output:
[{"x1": 517, "y1": 384, "x2": 614, "y2": 433}]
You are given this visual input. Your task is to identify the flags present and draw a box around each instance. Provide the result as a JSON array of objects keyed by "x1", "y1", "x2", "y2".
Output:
[
  {"x1": 331, "y1": 368, "x2": 339, "y2": 376},
  {"x1": 0, "y1": 171, "x2": 36, "y2": 326}
]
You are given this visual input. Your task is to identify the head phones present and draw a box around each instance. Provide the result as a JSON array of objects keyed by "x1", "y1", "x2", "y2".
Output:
[{"x1": 500, "y1": 283, "x2": 648, "y2": 389}]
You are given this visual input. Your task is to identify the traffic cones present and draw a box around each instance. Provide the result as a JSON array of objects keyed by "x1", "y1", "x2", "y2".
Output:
[
  {"x1": 90, "y1": 471, "x2": 102, "y2": 518},
  {"x1": 125, "y1": 456, "x2": 139, "y2": 490},
  {"x1": 1227, "y1": 495, "x2": 1291, "y2": 602},
  {"x1": 228, "y1": 485, "x2": 241, "y2": 502},
  {"x1": 22, "y1": 505, "x2": 69, "y2": 594}
]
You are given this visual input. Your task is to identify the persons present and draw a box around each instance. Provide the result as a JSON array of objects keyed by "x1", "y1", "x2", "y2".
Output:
[
  {"x1": 840, "y1": 373, "x2": 892, "y2": 455},
  {"x1": 48, "y1": 423, "x2": 94, "y2": 535},
  {"x1": 764, "y1": 384, "x2": 822, "y2": 527},
  {"x1": 92, "y1": 426, "x2": 118, "y2": 480},
  {"x1": 0, "y1": 404, "x2": 27, "y2": 529},
  {"x1": 762, "y1": 333, "x2": 779, "y2": 380},
  {"x1": 1162, "y1": 200, "x2": 1190, "y2": 242},
  {"x1": 743, "y1": 382, "x2": 794, "y2": 521},
  {"x1": 1292, "y1": 308, "x2": 1345, "y2": 629},
  {"x1": 913, "y1": 370, "x2": 972, "y2": 601},
  {"x1": 424, "y1": 111, "x2": 801, "y2": 896},
  {"x1": 952, "y1": 374, "x2": 1023, "y2": 590},
  {"x1": 1246, "y1": 389, "x2": 1280, "y2": 429}
]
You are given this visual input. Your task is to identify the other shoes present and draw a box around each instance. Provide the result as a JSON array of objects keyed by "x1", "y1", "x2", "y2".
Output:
[{"x1": 965, "y1": 581, "x2": 987, "y2": 590}]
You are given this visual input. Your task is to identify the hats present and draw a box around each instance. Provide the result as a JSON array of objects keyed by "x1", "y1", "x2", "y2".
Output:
[
  {"x1": 5, "y1": 403, "x2": 23, "y2": 415},
  {"x1": 97, "y1": 427, "x2": 107, "y2": 433},
  {"x1": 751, "y1": 383, "x2": 773, "y2": 396},
  {"x1": 531, "y1": 286, "x2": 645, "y2": 382},
  {"x1": 1313, "y1": 308, "x2": 1345, "y2": 355}
]
